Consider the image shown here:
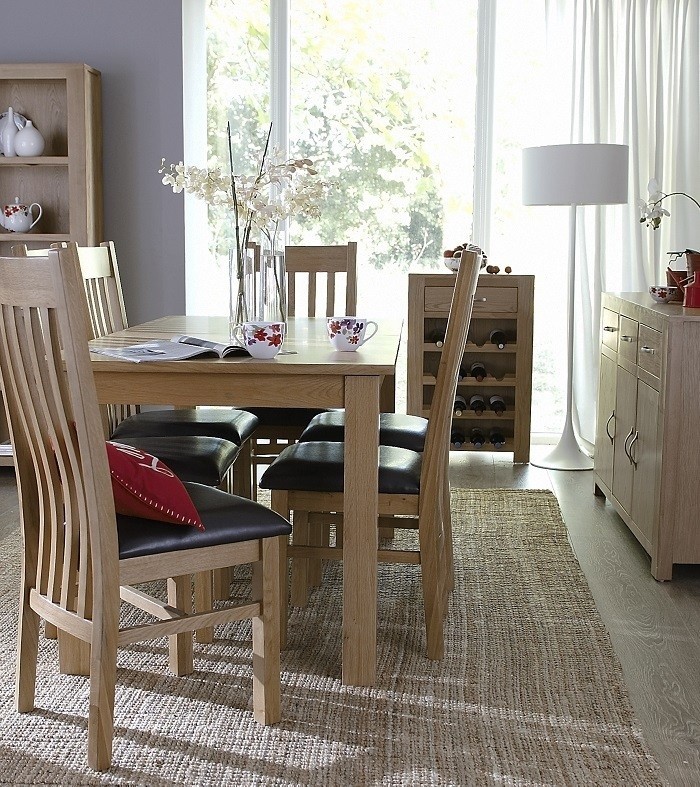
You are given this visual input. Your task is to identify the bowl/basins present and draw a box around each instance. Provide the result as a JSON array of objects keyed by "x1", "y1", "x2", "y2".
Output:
[
  {"x1": 444, "y1": 257, "x2": 487, "y2": 274},
  {"x1": 649, "y1": 286, "x2": 677, "y2": 304}
]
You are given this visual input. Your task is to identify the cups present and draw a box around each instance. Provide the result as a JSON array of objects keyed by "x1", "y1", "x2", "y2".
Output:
[
  {"x1": 327, "y1": 317, "x2": 378, "y2": 352},
  {"x1": 233, "y1": 321, "x2": 285, "y2": 360},
  {"x1": 666, "y1": 271, "x2": 686, "y2": 301}
]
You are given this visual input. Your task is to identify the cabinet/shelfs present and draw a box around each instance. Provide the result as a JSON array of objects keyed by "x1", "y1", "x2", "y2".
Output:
[
  {"x1": 592, "y1": 291, "x2": 700, "y2": 584},
  {"x1": 406, "y1": 272, "x2": 535, "y2": 465},
  {"x1": 0, "y1": 62, "x2": 104, "y2": 466}
]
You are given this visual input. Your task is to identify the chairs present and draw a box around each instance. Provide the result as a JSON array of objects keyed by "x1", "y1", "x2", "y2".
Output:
[{"x1": 0, "y1": 240, "x2": 482, "y2": 770}]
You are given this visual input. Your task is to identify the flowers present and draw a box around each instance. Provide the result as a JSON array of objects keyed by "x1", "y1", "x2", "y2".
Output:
[
  {"x1": 158, "y1": 156, "x2": 339, "y2": 322},
  {"x1": 635, "y1": 178, "x2": 700, "y2": 230}
]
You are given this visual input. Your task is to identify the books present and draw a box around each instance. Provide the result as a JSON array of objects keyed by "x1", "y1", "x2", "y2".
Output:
[{"x1": 88, "y1": 333, "x2": 251, "y2": 365}]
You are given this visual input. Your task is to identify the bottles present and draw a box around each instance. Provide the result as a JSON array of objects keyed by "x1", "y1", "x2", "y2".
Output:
[
  {"x1": 4, "y1": 106, "x2": 18, "y2": 158},
  {"x1": 471, "y1": 363, "x2": 486, "y2": 382},
  {"x1": 451, "y1": 429, "x2": 465, "y2": 447},
  {"x1": 470, "y1": 394, "x2": 486, "y2": 416},
  {"x1": 489, "y1": 394, "x2": 506, "y2": 417},
  {"x1": 15, "y1": 120, "x2": 45, "y2": 157},
  {"x1": 454, "y1": 395, "x2": 466, "y2": 417},
  {"x1": 490, "y1": 329, "x2": 507, "y2": 349},
  {"x1": 431, "y1": 328, "x2": 445, "y2": 348},
  {"x1": 458, "y1": 363, "x2": 467, "y2": 382},
  {"x1": 469, "y1": 427, "x2": 486, "y2": 448},
  {"x1": 488, "y1": 427, "x2": 505, "y2": 448}
]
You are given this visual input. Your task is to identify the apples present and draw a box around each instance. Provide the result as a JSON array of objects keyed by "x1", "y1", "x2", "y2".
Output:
[{"x1": 444, "y1": 243, "x2": 511, "y2": 274}]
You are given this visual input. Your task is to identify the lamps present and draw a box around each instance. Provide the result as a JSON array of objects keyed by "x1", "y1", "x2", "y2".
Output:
[{"x1": 523, "y1": 143, "x2": 631, "y2": 471}]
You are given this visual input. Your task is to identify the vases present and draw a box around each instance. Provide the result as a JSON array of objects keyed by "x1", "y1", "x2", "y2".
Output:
[
  {"x1": 4, "y1": 106, "x2": 19, "y2": 157},
  {"x1": 225, "y1": 226, "x2": 289, "y2": 346},
  {"x1": 14, "y1": 120, "x2": 46, "y2": 156}
]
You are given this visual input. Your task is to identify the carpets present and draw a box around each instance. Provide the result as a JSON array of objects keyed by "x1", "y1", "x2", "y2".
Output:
[{"x1": 1, "y1": 487, "x2": 672, "y2": 787}]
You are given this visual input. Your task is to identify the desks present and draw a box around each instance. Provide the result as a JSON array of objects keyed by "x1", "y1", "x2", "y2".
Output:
[{"x1": 43, "y1": 313, "x2": 406, "y2": 686}]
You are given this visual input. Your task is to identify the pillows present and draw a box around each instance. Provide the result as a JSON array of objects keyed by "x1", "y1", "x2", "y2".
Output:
[{"x1": 53, "y1": 423, "x2": 208, "y2": 531}]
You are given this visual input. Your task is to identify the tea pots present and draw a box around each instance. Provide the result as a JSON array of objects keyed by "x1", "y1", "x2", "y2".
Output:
[{"x1": 0, "y1": 196, "x2": 43, "y2": 233}]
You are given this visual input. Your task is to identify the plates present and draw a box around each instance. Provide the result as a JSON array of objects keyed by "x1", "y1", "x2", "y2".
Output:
[{"x1": 0, "y1": 112, "x2": 28, "y2": 157}]
